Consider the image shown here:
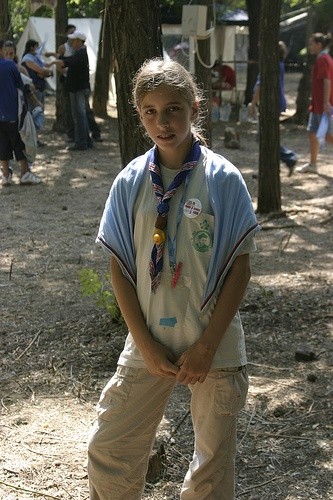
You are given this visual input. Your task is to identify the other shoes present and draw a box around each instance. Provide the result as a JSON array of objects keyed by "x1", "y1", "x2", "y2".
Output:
[
  {"x1": 298, "y1": 162, "x2": 316, "y2": 174},
  {"x1": 287, "y1": 159, "x2": 296, "y2": 177}
]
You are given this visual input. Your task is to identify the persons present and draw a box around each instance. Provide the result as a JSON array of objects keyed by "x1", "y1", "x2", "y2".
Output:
[
  {"x1": 57, "y1": 24, "x2": 102, "y2": 143},
  {"x1": 0, "y1": 40, "x2": 42, "y2": 186},
  {"x1": 247, "y1": 40, "x2": 299, "y2": 184},
  {"x1": 295, "y1": 32, "x2": 333, "y2": 174},
  {"x1": 43, "y1": 31, "x2": 94, "y2": 152},
  {"x1": 87, "y1": 57, "x2": 263, "y2": 500},
  {"x1": 21, "y1": 40, "x2": 54, "y2": 147},
  {"x1": 211, "y1": 64, "x2": 236, "y2": 97},
  {"x1": 2, "y1": 41, "x2": 44, "y2": 181}
]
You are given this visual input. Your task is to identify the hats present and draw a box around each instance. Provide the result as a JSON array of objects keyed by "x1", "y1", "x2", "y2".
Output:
[{"x1": 68, "y1": 30, "x2": 87, "y2": 42}]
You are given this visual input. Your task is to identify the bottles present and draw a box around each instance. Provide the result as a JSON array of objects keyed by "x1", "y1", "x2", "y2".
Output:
[{"x1": 239, "y1": 104, "x2": 248, "y2": 123}]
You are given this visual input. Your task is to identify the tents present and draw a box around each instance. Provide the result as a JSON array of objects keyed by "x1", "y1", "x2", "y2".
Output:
[{"x1": 15, "y1": 16, "x2": 105, "y2": 74}]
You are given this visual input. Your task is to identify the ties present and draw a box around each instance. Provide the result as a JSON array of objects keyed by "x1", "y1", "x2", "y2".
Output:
[{"x1": 149, "y1": 131, "x2": 201, "y2": 293}]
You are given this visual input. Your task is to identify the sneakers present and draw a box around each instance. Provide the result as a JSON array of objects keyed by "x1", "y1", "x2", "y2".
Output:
[
  {"x1": 0, "y1": 166, "x2": 14, "y2": 185},
  {"x1": 19, "y1": 171, "x2": 43, "y2": 184}
]
